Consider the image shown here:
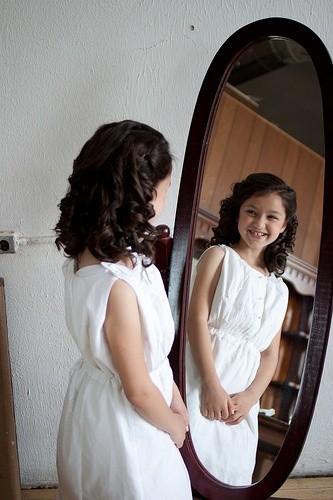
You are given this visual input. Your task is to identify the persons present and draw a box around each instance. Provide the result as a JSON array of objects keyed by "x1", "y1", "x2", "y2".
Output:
[
  {"x1": 184, "y1": 171, "x2": 297, "y2": 486},
  {"x1": 53, "y1": 120, "x2": 193, "y2": 500}
]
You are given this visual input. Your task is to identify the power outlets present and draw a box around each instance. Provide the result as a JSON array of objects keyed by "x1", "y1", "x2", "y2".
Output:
[{"x1": 0, "y1": 233, "x2": 17, "y2": 253}]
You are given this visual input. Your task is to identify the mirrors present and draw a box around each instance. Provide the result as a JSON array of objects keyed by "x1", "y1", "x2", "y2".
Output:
[{"x1": 166, "y1": 18, "x2": 332, "y2": 499}]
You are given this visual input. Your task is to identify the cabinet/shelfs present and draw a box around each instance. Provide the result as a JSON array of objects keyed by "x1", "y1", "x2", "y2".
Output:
[{"x1": 188, "y1": 81, "x2": 325, "y2": 487}]
